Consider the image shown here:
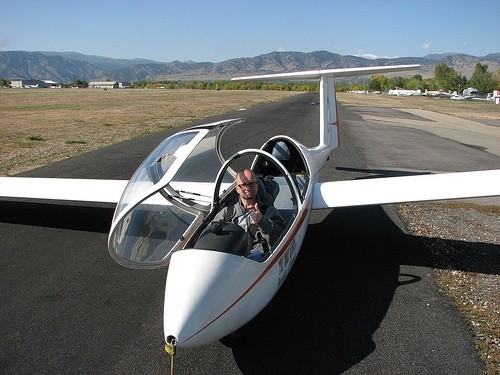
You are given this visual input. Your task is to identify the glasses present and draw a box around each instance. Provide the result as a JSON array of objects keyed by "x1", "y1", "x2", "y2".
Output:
[{"x1": 238, "y1": 181, "x2": 258, "y2": 188}]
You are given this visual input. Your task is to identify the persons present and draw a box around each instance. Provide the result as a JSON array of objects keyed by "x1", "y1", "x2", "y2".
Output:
[{"x1": 210, "y1": 169, "x2": 285, "y2": 259}]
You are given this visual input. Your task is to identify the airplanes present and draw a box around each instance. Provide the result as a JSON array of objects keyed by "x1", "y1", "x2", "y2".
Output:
[{"x1": 0, "y1": 62, "x2": 500, "y2": 356}]
